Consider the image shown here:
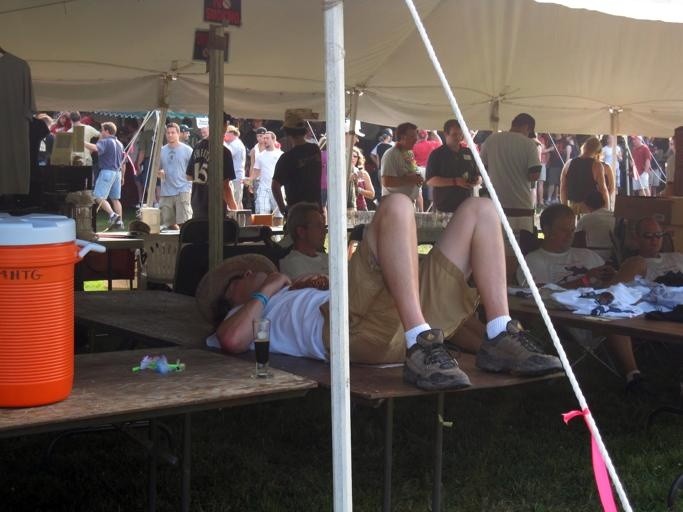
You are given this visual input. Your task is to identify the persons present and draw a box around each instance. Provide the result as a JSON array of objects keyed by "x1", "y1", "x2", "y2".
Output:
[
  {"x1": 534, "y1": 133, "x2": 676, "y2": 212},
  {"x1": 345, "y1": 111, "x2": 543, "y2": 232},
  {"x1": 195, "y1": 193, "x2": 564, "y2": 393},
  {"x1": 516, "y1": 203, "x2": 657, "y2": 399},
  {"x1": 31, "y1": 109, "x2": 327, "y2": 231},
  {"x1": 574, "y1": 191, "x2": 616, "y2": 264},
  {"x1": 624, "y1": 216, "x2": 683, "y2": 283},
  {"x1": 277, "y1": 202, "x2": 328, "y2": 277}
]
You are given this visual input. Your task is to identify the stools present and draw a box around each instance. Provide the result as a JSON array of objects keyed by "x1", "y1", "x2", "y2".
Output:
[{"x1": 348, "y1": 225, "x2": 445, "y2": 265}]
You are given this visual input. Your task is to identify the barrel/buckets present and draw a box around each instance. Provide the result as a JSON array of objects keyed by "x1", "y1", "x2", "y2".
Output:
[{"x1": 0, "y1": 209, "x2": 106, "y2": 408}]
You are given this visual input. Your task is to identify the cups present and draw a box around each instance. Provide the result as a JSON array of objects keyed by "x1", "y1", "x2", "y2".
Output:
[
  {"x1": 252, "y1": 318, "x2": 271, "y2": 379},
  {"x1": 272, "y1": 214, "x2": 282, "y2": 228},
  {"x1": 238, "y1": 214, "x2": 246, "y2": 227},
  {"x1": 415, "y1": 212, "x2": 453, "y2": 226},
  {"x1": 348, "y1": 211, "x2": 376, "y2": 224}
]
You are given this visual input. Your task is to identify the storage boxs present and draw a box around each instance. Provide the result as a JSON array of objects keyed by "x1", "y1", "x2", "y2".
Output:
[{"x1": 614, "y1": 194, "x2": 683, "y2": 253}]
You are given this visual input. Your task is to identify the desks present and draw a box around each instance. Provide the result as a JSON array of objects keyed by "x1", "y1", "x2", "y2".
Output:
[
  {"x1": 270, "y1": 226, "x2": 444, "y2": 261},
  {"x1": 505, "y1": 281, "x2": 683, "y2": 386},
  {"x1": 76, "y1": 291, "x2": 568, "y2": 512},
  {"x1": 79, "y1": 233, "x2": 144, "y2": 293},
  {"x1": 1, "y1": 350, "x2": 319, "y2": 511},
  {"x1": 157, "y1": 226, "x2": 282, "y2": 275}
]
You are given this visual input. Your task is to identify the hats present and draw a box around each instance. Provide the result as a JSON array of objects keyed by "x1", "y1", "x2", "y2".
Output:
[
  {"x1": 517, "y1": 113, "x2": 537, "y2": 138},
  {"x1": 180, "y1": 126, "x2": 193, "y2": 132},
  {"x1": 196, "y1": 254, "x2": 276, "y2": 321},
  {"x1": 257, "y1": 127, "x2": 266, "y2": 134},
  {"x1": 345, "y1": 119, "x2": 365, "y2": 138},
  {"x1": 280, "y1": 116, "x2": 306, "y2": 132}
]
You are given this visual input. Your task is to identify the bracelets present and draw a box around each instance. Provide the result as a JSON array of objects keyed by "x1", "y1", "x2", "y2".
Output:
[{"x1": 251, "y1": 292, "x2": 268, "y2": 306}]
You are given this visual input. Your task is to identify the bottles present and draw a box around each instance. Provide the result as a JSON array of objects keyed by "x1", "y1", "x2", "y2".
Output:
[{"x1": 72, "y1": 204, "x2": 93, "y2": 240}]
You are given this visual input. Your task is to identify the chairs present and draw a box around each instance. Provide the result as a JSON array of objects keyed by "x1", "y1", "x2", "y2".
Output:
[{"x1": 174, "y1": 215, "x2": 239, "y2": 296}]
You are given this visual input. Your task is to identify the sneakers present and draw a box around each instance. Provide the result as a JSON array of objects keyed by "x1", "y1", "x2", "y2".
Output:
[
  {"x1": 405, "y1": 328, "x2": 472, "y2": 391},
  {"x1": 475, "y1": 319, "x2": 562, "y2": 377},
  {"x1": 116, "y1": 221, "x2": 124, "y2": 230},
  {"x1": 627, "y1": 374, "x2": 648, "y2": 391},
  {"x1": 107, "y1": 215, "x2": 120, "y2": 229}
]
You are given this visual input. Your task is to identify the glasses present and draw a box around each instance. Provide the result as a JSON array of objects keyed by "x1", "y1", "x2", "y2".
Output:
[{"x1": 641, "y1": 232, "x2": 662, "y2": 238}]
